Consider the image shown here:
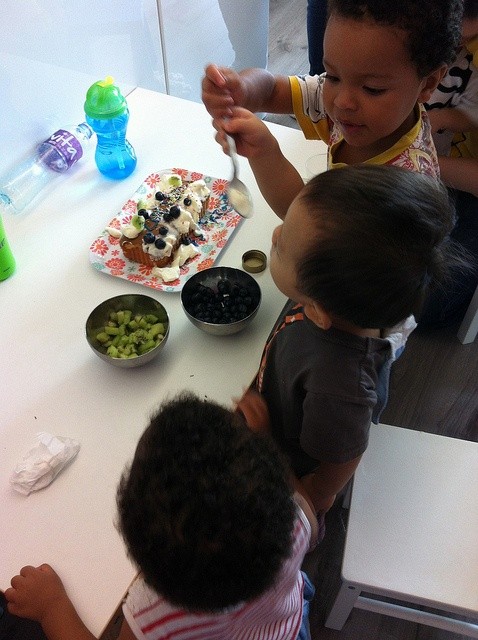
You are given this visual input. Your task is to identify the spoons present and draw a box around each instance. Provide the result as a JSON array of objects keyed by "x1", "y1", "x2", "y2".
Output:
[{"x1": 221, "y1": 108, "x2": 256, "y2": 220}]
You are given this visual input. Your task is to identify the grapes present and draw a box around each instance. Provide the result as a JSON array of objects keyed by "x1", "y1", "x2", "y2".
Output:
[{"x1": 185, "y1": 280, "x2": 255, "y2": 325}]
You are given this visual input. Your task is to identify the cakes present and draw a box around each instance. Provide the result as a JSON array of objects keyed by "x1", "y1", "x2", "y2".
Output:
[{"x1": 106, "y1": 174, "x2": 211, "y2": 284}]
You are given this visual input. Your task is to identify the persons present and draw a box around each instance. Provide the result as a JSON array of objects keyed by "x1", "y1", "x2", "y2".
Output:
[
  {"x1": 423, "y1": 46, "x2": 478, "y2": 156},
  {"x1": 212, "y1": 103, "x2": 457, "y2": 555},
  {"x1": 416, "y1": 0, "x2": 478, "y2": 330},
  {"x1": 4, "y1": 386, "x2": 319, "y2": 640},
  {"x1": 204, "y1": 0, "x2": 463, "y2": 364}
]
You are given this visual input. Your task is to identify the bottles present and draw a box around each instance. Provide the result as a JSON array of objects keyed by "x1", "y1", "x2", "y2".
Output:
[
  {"x1": 83, "y1": 74, "x2": 139, "y2": 184},
  {"x1": 0, "y1": 120, "x2": 97, "y2": 219}
]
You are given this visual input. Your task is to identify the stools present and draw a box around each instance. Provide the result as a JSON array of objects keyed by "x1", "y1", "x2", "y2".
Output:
[{"x1": 325, "y1": 422, "x2": 478, "y2": 640}]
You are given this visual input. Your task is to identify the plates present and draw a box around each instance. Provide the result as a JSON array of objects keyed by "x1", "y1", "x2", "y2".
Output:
[{"x1": 86, "y1": 166, "x2": 244, "y2": 294}]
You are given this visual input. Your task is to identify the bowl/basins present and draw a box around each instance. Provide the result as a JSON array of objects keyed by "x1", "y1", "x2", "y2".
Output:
[
  {"x1": 83, "y1": 293, "x2": 171, "y2": 369},
  {"x1": 180, "y1": 265, "x2": 264, "y2": 337}
]
